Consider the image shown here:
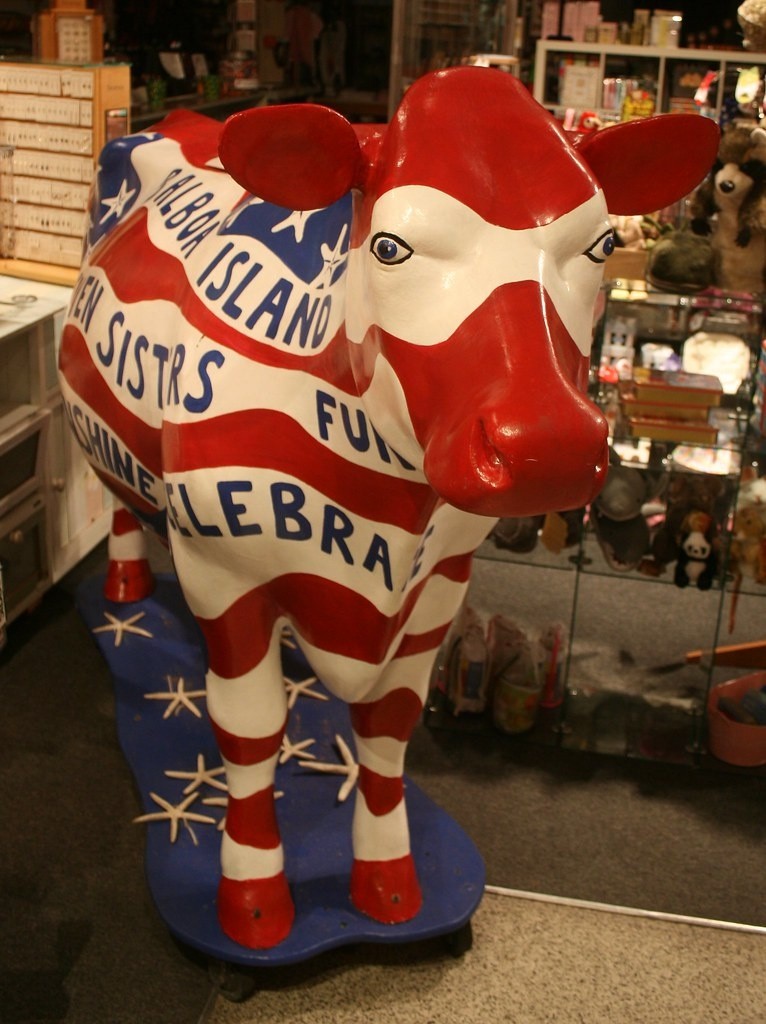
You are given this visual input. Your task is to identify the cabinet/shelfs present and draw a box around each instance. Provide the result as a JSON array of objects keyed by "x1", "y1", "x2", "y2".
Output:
[
  {"x1": 0, "y1": 55, "x2": 131, "y2": 287},
  {"x1": 403, "y1": 276, "x2": 766, "y2": 937},
  {"x1": 1, "y1": 274, "x2": 113, "y2": 648},
  {"x1": 536, "y1": 41, "x2": 766, "y2": 228}
]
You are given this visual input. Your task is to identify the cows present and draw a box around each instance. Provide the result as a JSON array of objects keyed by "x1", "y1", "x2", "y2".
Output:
[{"x1": 57, "y1": 62, "x2": 716, "y2": 954}]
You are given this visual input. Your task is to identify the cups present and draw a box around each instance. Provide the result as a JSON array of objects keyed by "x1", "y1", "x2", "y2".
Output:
[
  {"x1": 651, "y1": 10, "x2": 681, "y2": 48},
  {"x1": 148, "y1": 79, "x2": 166, "y2": 106},
  {"x1": 204, "y1": 77, "x2": 219, "y2": 96}
]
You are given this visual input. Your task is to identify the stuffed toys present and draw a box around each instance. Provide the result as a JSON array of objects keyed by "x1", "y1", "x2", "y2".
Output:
[
  {"x1": 674, "y1": 530, "x2": 722, "y2": 590},
  {"x1": 422, "y1": 121, "x2": 766, "y2": 592}
]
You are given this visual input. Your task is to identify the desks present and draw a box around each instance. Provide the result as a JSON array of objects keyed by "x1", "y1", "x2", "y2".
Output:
[{"x1": 132, "y1": 84, "x2": 321, "y2": 135}]
